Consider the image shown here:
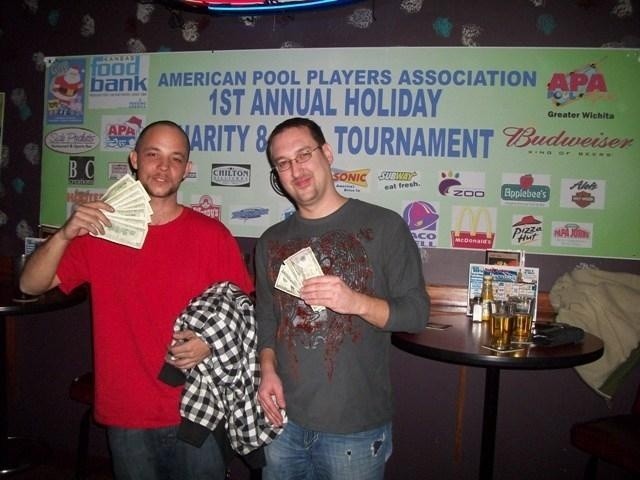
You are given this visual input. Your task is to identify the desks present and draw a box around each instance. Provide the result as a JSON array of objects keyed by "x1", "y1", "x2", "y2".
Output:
[
  {"x1": 386, "y1": 311, "x2": 607, "y2": 480},
  {"x1": 0, "y1": 259, "x2": 92, "y2": 480}
]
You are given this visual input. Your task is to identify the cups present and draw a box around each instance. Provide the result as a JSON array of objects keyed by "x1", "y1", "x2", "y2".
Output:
[
  {"x1": 12, "y1": 254, "x2": 34, "y2": 303},
  {"x1": 509, "y1": 296, "x2": 534, "y2": 314},
  {"x1": 511, "y1": 311, "x2": 533, "y2": 346},
  {"x1": 490, "y1": 313, "x2": 513, "y2": 349}
]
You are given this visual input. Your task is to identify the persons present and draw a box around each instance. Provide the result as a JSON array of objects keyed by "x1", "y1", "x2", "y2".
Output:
[
  {"x1": 18, "y1": 122, "x2": 256, "y2": 480},
  {"x1": 255, "y1": 119, "x2": 431, "y2": 480}
]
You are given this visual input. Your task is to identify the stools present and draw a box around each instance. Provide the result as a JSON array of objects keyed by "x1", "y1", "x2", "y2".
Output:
[
  {"x1": 69, "y1": 367, "x2": 113, "y2": 479},
  {"x1": 569, "y1": 412, "x2": 640, "y2": 480}
]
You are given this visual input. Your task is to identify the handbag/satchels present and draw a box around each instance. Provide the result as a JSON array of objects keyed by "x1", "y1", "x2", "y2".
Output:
[{"x1": 532, "y1": 321, "x2": 585, "y2": 347}]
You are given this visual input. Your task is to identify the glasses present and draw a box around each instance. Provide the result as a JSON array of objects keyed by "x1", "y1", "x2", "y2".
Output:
[{"x1": 276, "y1": 145, "x2": 321, "y2": 172}]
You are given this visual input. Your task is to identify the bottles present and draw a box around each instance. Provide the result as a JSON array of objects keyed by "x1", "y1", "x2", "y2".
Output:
[
  {"x1": 480, "y1": 274, "x2": 493, "y2": 320},
  {"x1": 492, "y1": 284, "x2": 509, "y2": 315},
  {"x1": 472, "y1": 297, "x2": 482, "y2": 322}
]
